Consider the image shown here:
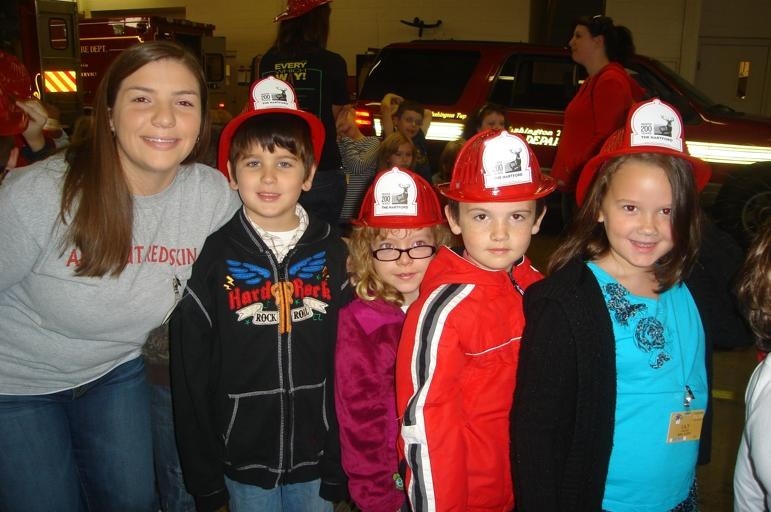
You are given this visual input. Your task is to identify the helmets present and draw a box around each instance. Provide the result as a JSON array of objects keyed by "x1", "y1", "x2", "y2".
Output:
[
  {"x1": 0, "y1": 54, "x2": 34, "y2": 138},
  {"x1": 217, "y1": 73, "x2": 325, "y2": 178},
  {"x1": 576, "y1": 99, "x2": 712, "y2": 207},
  {"x1": 438, "y1": 128, "x2": 558, "y2": 202},
  {"x1": 273, "y1": 0, "x2": 328, "y2": 22},
  {"x1": 350, "y1": 166, "x2": 443, "y2": 229}
]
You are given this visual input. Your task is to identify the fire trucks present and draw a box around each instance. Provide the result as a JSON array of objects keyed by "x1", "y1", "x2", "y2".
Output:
[
  {"x1": 50, "y1": 10, "x2": 227, "y2": 144},
  {"x1": 0, "y1": 0, "x2": 82, "y2": 148}
]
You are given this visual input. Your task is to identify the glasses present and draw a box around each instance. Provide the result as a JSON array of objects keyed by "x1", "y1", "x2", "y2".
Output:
[{"x1": 369, "y1": 244, "x2": 435, "y2": 261}]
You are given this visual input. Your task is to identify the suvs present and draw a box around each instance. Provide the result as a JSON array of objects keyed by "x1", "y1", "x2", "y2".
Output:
[{"x1": 349, "y1": 37, "x2": 770, "y2": 242}]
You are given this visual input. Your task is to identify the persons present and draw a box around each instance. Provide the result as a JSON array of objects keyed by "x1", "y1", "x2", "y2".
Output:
[
  {"x1": 509, "y1": 97, "x2": 715, "y2": 511},
  {"x1": 166, "y1": 76, "x2": 353, "y2": 510},
  {"x1": 732, "y1": 190, "x2": 770, "y2": 511},
  {"x1": 330, "y1": 93, "x2": 504, "y2": 232},
  {"x1": 393, "y1": 127, "x2": 556, "y2": 511},
  {"x1": 334, "y1": 167, "x2": 453, "y2": 512},
  {"x1": 549, "y1": 14, "x2": 645, "y2": 249},
  {"x1": 257, "y1": 1, "x2": 355, "y2": 223},
  {"x1": 13, "y1": 99, "x2": 56, "y2": 166},
  {"x1": 0, "y1": 43, "x2": 247, "y2": 511}
]
